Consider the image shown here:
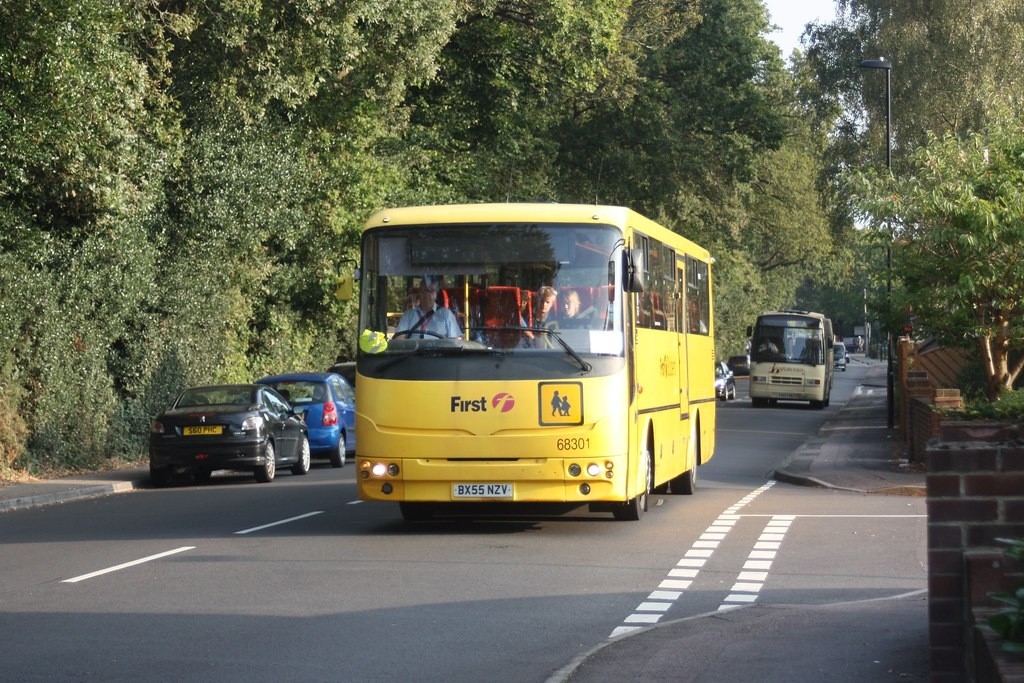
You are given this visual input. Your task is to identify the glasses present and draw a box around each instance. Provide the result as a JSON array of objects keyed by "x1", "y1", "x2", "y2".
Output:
[{"x1": 419, "y1": 287, "x2": 436, "y2": 294}]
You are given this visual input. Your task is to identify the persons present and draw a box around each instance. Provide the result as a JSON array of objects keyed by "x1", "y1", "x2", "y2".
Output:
[
  {"x1": 395, "y1": 277, "x2": 463, "y2": 341},
  {"x1": 518, "y1": 288, "x2": 535, "y2": 348},
  {"x1": 532, "y1": 285, "x2": 557, "y2": 332},
  {"x1": 800, "y1": 339, "x2": 818, "y2": 361},
  {"x1": 758, "y1": 336, "x2": 778, "y2": 352},
  {"x1": 559, "y1": 290, "x2": 590, "y2": 319},
  {"x1": 856, "y1": 335, "x2": 864, "y2": 351}
]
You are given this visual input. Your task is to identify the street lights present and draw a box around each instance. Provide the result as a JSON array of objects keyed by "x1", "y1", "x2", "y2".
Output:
[{"x1": 861, "y1": 60, "x2": 895, "y2": 429}]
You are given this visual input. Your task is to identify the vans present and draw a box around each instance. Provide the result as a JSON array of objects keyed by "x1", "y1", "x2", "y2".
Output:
[{"x1": 833, "y1": 342, "x2": 850, "y2": 371}]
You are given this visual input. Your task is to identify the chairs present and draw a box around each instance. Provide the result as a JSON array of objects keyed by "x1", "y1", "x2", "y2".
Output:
[{"x1": 409, "y1": 285, "x2": 702, "y2": 350}]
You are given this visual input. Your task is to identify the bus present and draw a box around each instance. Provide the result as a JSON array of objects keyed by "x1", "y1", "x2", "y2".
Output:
[
  {"x1": 336, "y1": 202, "x2": 716, "y2": 522},
  {"x1": 746, "y1": 309, "x2": 834, "y2": 409}
]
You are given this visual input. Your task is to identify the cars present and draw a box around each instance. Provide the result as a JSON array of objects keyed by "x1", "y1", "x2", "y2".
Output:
[
  {"x1": 148, "y1": 384, "x2": 311, "y2": 487},
  {"x1": 253, "y1": 362, "x2": 357, "y2": 468},
  {"x1": 714, "y1": 362, "x2": 736, "y2": 401},
  {"x1": 727, "y1": 355, "x2": 750, "y2": 376}
]
